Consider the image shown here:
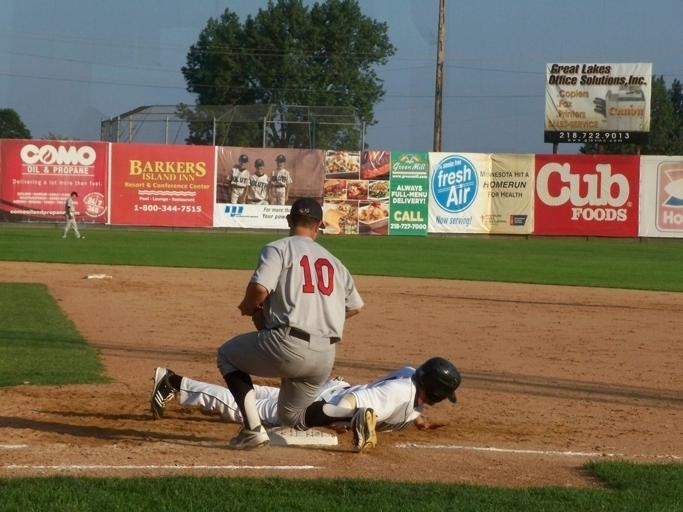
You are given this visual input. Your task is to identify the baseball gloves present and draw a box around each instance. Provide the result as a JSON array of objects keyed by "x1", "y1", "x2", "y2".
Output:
[{"x1": 251, "y1": 303, "x2": 267, "y2": 330}]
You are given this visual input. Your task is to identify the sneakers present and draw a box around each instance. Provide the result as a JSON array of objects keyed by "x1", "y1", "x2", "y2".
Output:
[
  {"x1": 230, "y1": 424, "x2": 271, "y2": 449},
  {"x1": 349, "y1": 408, "x2": 378, "y2": 453},
  {"x1": 151, "y1": 367, "x2": 178, "y2": 420}
]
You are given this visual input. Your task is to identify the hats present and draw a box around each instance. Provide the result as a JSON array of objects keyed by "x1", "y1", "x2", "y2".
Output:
[
  {"x1": 290, "y1": 198, "x2": 325, "y2": 230},
  {"x1": 71, "y1": 192, "x2": 78, "y2": 197}
]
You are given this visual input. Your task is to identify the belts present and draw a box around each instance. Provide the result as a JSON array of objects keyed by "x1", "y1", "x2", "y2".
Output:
[{"x1": 281, "y1": 325, "x2": 340, "y2": 344}]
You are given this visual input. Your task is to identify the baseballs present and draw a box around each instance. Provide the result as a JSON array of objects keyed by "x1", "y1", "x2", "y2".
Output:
[{"x1": 82, "y1": 236, "x2": 85, "y2": 240}]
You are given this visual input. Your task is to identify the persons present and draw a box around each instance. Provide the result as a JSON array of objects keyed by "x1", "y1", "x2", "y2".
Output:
[
  {"x1": 225, "y1": 154, "x2": 250, "y2": 205},
  {"x1": 61, "y1": 192, "x2": 86, "y2": 240},
  {"x1": 247, "y1": 159, "x2": 270, "y2": 205},
  {"x1": 269, "y1": 154, "x2": 292, "y2": 205},
  {"x1": 217, "y1": 196, "x2": 378, "y2": 452},
  {"x1": 150, "y1": 356, "x2": 461, "y2": 434}
]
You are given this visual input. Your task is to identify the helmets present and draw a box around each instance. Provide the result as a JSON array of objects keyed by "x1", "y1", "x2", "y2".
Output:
[
  {"x1": 255, "y1": 159, "x2": 264, "y2": 167},
  {"x1": 275, "y1": 155, "x2": 285, "y2": 162},
  {"x1": 416, "y1": 358, "x2": 460, "y2": 403},
  {"x1": 240, "y1": 155, "x2": 249, "y2": 162}
]
